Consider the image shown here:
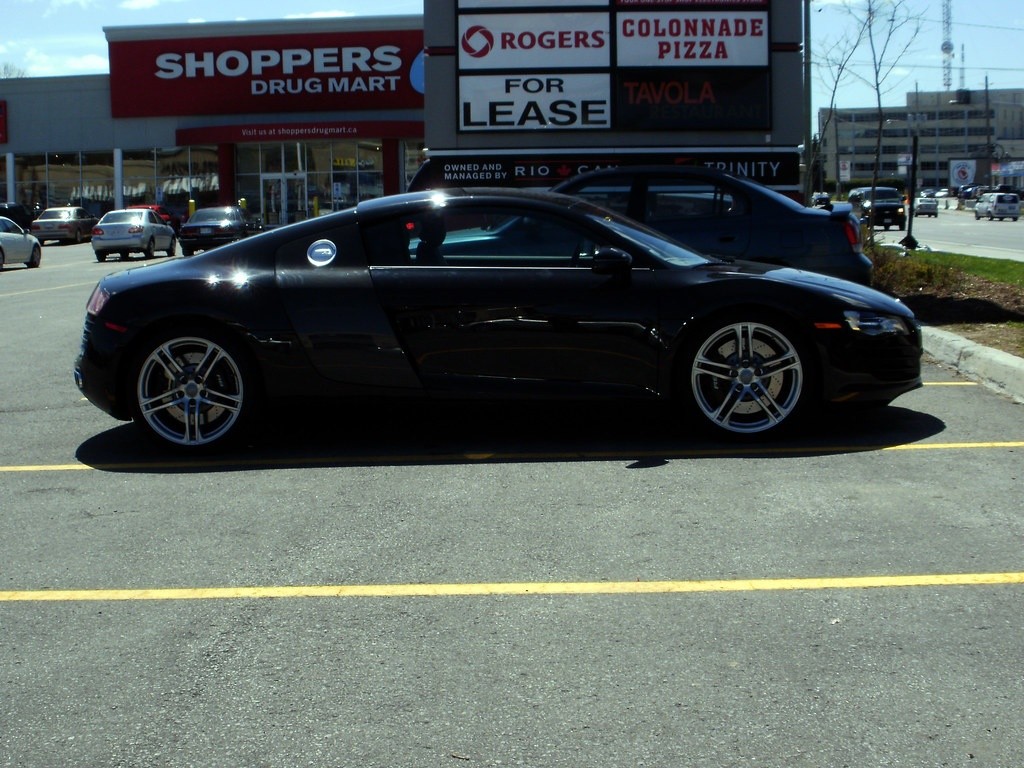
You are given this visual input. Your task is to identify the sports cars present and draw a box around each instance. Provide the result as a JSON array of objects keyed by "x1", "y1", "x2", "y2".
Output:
[{"x1": 74, "y1": 189, "x2": 925, "y2": 458}]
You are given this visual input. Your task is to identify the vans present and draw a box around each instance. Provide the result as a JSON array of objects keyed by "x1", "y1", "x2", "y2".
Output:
[
  {"x1": 848, "y1": 186, "x2": 906, "y2": 231},
  {"x1": 0, "y1": 200, "x2": 40, "y2": 234},
  {"x1": 974, "y1": 191, "x2": 1021, "y2": 222}
]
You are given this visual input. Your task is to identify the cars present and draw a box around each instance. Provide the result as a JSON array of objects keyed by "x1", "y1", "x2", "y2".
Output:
[
  {"x1": 812, "y1": 191, "x2": 830, "y2": 207},
  {"x1": 91, "y1": 208, "x2": 176, "y2": 262},
  {"x1": 913, "y1": 196, "x2": 938, "y2": 217},
  {"x1": 0, "y1": 215, "x2": 42, "y2": 270},
  {"x1": 179, "y1": 204, "x2": 267, "y2": 258},
  {"x1": 128, "y1": 203, "x2": 178, "y2": 233},
  {"x1": 409, "y1": 162, "x2": 874, "y2": 294},
  {"x1": 920, "y1": 182, "x2": 1024, "y2": 201},
  {"x1": 31, "y1": 206, "x2": 98, "y2": 246}
]
